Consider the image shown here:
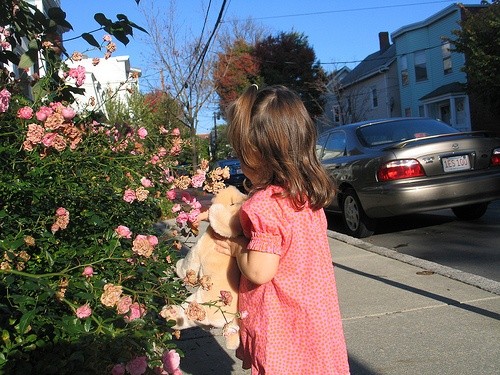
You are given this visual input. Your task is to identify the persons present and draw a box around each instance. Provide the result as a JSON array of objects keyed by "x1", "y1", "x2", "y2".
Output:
[{"x1": 191, "y1": 81, "x2": 352, "y2": 373}]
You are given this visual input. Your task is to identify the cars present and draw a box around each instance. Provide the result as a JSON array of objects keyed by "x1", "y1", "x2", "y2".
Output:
[
  {"x1": 216, "y1": 157, "x2": 252, "y2": 194},
  {"x1": 317, "y1": 117, "x2": 500, "y2": 239}
]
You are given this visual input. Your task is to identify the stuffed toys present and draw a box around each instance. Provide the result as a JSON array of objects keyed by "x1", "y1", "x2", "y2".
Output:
[{"x1": 162, "y1": 185, "x2": 242, "y2": 351}]
